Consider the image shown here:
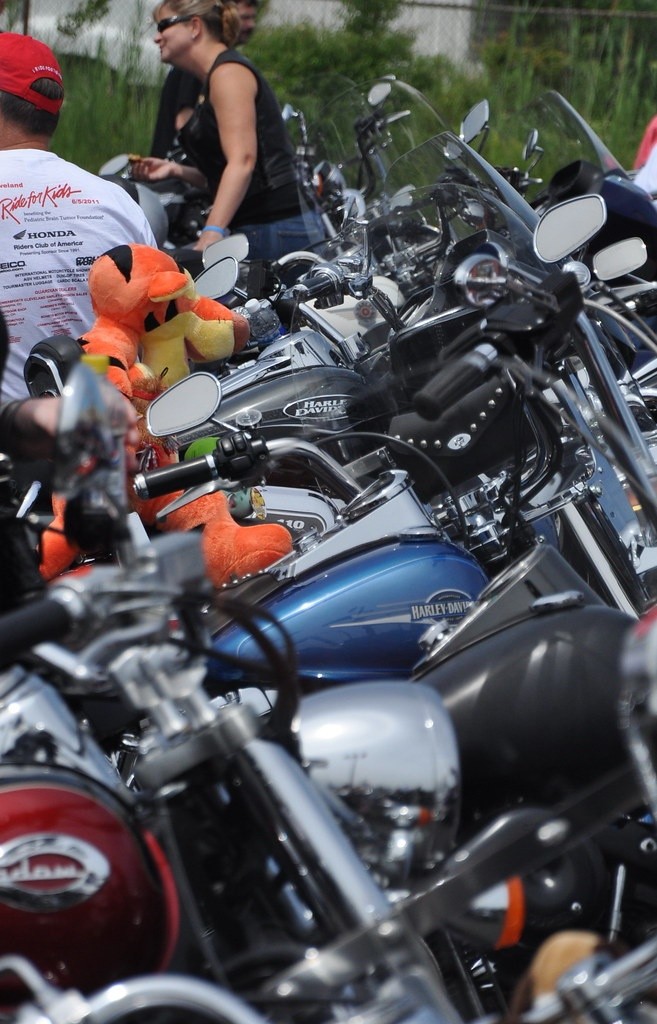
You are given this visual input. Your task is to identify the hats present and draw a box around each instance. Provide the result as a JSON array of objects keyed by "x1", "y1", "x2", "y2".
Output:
[{"x1": 0, "y1": 32, "x2": 64, "y2": 114}]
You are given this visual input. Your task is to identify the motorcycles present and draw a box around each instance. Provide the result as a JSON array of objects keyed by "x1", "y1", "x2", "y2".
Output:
[{"x1": 0, "y1": 73, "x2": 655, "y2": 1024}]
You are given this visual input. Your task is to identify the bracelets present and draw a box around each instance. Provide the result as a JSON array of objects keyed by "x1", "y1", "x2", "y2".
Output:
[
  {"x1": 0, "y1": 400, "x2": 27, "y2": 455},
  {"x1": 202, "y1": 226, "x2": 225, "y2": 237}
]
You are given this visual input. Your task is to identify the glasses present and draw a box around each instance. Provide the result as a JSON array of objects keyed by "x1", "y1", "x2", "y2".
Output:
[{"x1": 158, "y1": 14, "x2": 211, "y2": 33}]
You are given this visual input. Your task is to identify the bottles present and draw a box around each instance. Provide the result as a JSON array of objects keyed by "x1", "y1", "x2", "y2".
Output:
[
  {"x1": 245, "y1": 298, "x2": 286, "y2": 349},
  {"x1": 80, "y1": 354, "x2": 129, "y2": 514}
]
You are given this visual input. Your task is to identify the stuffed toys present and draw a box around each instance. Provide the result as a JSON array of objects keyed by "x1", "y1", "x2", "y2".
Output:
[{"x1": 37, "y1": 242, "x2": 293, "y2": 590}]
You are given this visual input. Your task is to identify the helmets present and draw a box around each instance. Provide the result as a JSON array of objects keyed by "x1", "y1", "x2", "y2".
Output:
[{"x1": 0, "y1": 759, "x2": 179, "y2": 1013}]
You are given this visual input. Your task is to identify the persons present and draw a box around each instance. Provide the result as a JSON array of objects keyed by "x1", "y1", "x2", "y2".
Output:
[
  {"x1": 0, "y1": 30, "x2": 158, "y2": 403},
  {"x1": 131, "y1": 0, "x2": 321, "y2": 288},
  {"x1": 0, "y1": 374, "x2": 141, "y2": 480}
]
